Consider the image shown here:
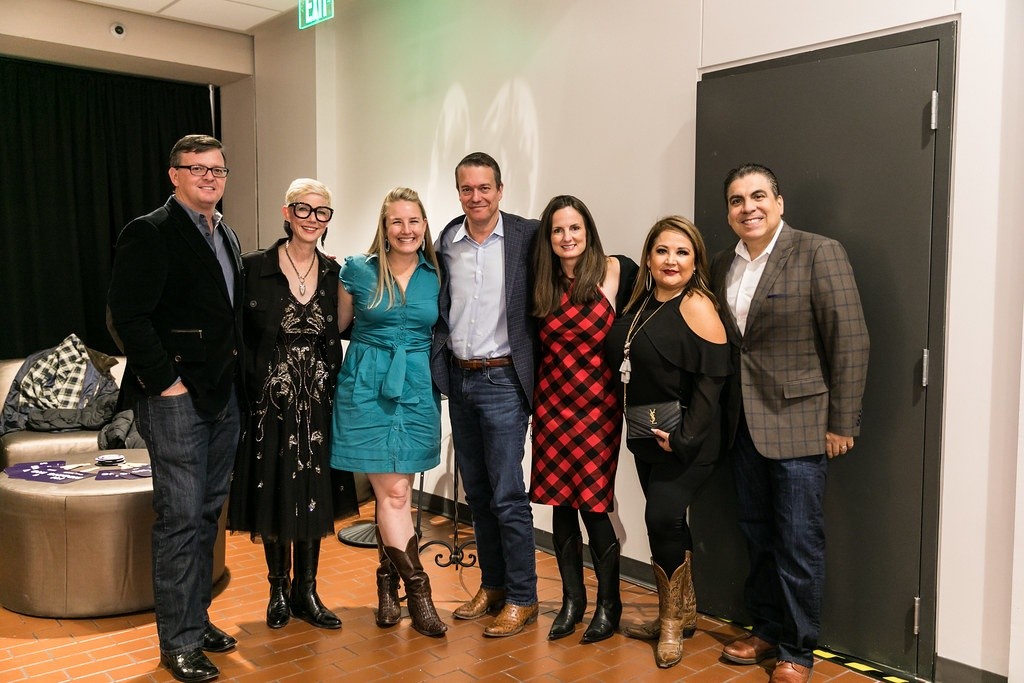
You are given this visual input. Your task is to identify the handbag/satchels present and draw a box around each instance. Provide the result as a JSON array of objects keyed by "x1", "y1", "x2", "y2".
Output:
[{"x1": 625, "y1": 401, "x2": 683, "y2": 439}]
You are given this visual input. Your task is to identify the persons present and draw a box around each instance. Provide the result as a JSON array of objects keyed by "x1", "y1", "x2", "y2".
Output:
[
  {"x1": 527, "y1": 195, "x2": 640, "y2": 643},
  {"x1": 225, "y1": 178, "x2": 344, "y2": 628},
  {"x1": 108, "y1": 134, "x2": 336, "y2": 683},
  {"x1": 616, "y1": 215, "x2": 730, "y2": 667},
  {"x1": 426, "y1": 151, "x2": 541, "y2": 637},
  {"x1": 707, "y1": 163, "x2": 869, "y2": 683},
  {"x1": 331, "y1": 187, "x2": 448, "y2": 636}
]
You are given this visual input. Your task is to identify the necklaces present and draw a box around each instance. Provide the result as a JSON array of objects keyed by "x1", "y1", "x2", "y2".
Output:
[
  {"x1": 620, "y1": 286, "x2": 676, "y2": 385},
  {"x1": 285, "y1": 241, "x2": 316, "y2": 296}
]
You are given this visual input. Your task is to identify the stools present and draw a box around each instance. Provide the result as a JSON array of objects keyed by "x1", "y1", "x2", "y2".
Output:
[{"x1": 0, "y1": 446, "x2": 228, "y2": 619}]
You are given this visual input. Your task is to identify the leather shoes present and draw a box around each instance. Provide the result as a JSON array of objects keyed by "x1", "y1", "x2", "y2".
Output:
[
  {"x1": 160, "y1": 644, "x2": 220, "y2": 682},
  {"x1": 484, "y1": 599, "x2": 539, "y2": 636},
  {"x1": 454, "y1": 586, "x2": 507, "y2": 618},
  {"x1": 769, "y1": 659, "x2": 814, "y2": 683},
  {"x1": 199, "y1": 621, "x2": 237, "y2": 650},
  {"x1": 720, "y1": 636, "x2": 774, "y2": 665}
]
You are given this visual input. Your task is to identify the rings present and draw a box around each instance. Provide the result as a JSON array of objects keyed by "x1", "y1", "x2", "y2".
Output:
[{"x1": 841, "y1": 446, "x2": 846, "y2": 449}]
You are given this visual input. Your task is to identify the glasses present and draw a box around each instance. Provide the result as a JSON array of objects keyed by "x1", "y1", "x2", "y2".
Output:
[
  {"x1": 288, "y1": 202, "x2": 334, "y2": 222},
  {"x1": 172, "y1": 164, "x2": 230, "y2": 178}
]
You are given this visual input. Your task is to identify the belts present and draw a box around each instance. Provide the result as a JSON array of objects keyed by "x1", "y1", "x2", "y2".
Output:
[{"x1": 451, "y1": 355, "x2": 513, "y2": 369}]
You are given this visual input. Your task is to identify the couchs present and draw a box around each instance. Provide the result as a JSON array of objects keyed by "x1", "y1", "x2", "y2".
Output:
[{"x1": 0, "y1": 355, "x2": 126, "y2": 468}]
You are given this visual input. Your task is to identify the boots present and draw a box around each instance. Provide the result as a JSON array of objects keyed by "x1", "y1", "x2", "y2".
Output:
[
  {"x1": 648, "y1": 550, "x2": 692, "y2": 666},
  {"x1": 583, "y1": 541, "x2": 621, "y2": 642},
  {"x1": 381, "y1": 532, "x2": 447, "y2": 636},
  {"x1": 549, "y1": 532, "x2": 587, "y2": 639},
  {"x1": 289, "y1": 531, "x2": 342, "y2": 629},
  {"x1": 374, "y1": 525, "x2": 402, "y2": 624},
  {"x1": 624, "y1": 552, "x2": 693, "y2": 639},
  {"x1": 262, "y1": 527, "x2": 291, "y2": 627}
]
